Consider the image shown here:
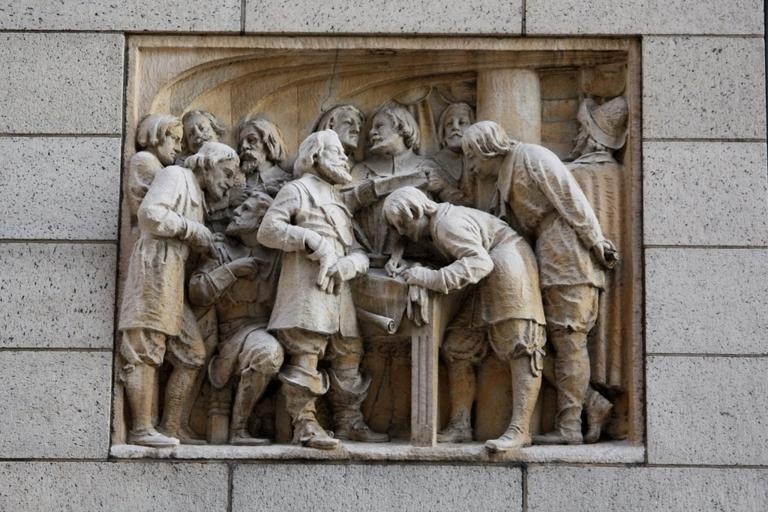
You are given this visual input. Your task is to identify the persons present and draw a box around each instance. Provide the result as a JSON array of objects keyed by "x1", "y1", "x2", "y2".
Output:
[{"x1": 119, "y1": 98, "x2": 630, "y2": 453}]
